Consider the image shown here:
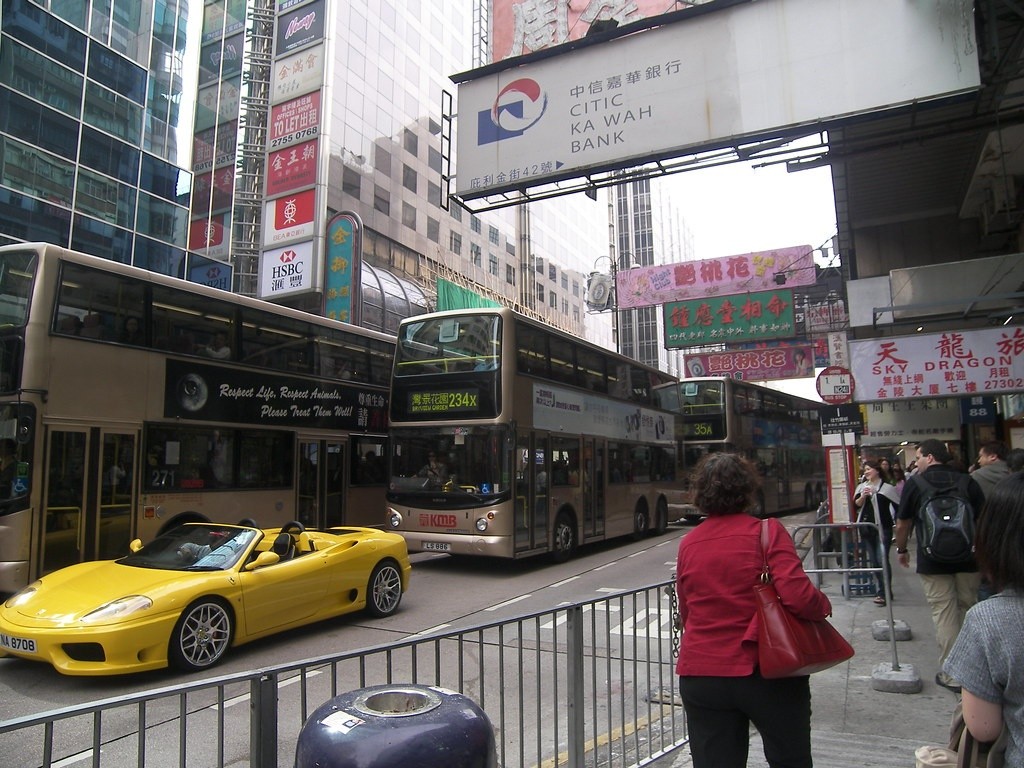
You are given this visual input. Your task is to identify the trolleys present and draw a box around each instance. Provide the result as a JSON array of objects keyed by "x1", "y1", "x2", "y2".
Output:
[{"x1": 841, "y1": 527, "x2": 877, "y2": 596}]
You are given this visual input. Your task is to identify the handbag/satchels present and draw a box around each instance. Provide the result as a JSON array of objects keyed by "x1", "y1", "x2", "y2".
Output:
[{"x1": 754, "y1": 517, "x2": 856, "y2": 679}]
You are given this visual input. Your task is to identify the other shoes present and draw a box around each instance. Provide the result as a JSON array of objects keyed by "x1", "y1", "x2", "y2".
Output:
[
  {"x1": 874, "y1": 591, "x2": 895, "y2": 605},
  {"x1": 935, "y1": 673, "x2": 962, "y2": 694}
]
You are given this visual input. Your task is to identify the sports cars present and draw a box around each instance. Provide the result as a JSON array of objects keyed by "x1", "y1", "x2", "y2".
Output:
[{"x1": 0, "y1": 519, "x2": 412, "y2": 676}]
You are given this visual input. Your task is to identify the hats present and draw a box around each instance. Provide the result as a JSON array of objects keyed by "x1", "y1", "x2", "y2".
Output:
[{"x1": 208, "y1": 530, "x2": 228, "y2": 537}]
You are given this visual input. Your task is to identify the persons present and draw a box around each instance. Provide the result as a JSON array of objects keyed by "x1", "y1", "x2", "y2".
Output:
[
  {"x1": 180, "y1": 528, "x2": 235, "y2": 566},
  {"x1": 676, "y1": 450, "x2": 832, "y2": 768},
  {"x1": 792, "y1": 348, "x2": 808, "y2": 375},
  {"x1": 472, "y1": 346, "x2": 500, "y2": 371},
  {"x1": 412, "y1": 451, "x2": 448, "y2": 484},
  {"x1": 108, "y1": 459, "x2": 127, "y2": 493},
  {"x1": 941, "y1": 475, "x2": 1024, "y2": 768},
  {"x1": 853, "y1": 460, "x2": 901, "y2": 604},
  {"x1": 0, "y1": 438, "x2": 402, "y2": 489},
  {"x1": 896, "y1": 438, "x2": 986, "y2": 693},
  {"x1": 536, "y1": 463, "x2": 623, "y2": 521},
  {"x1": 858, "y1": 440, "x2": 1024, "y2": 603},
  {"x1": 121, "y1": 317, "x2": 371, "y2": 382}
]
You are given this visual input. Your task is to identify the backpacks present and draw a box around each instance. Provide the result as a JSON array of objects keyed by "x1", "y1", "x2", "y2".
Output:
[{"x1": 910, "y1": 469, "x2": 980, "y2": 569}]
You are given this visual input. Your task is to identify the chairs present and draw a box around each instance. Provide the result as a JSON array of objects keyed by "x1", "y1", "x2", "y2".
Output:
[{"x1": 254, "y1": 533, "x2": 298, "y2": 568}]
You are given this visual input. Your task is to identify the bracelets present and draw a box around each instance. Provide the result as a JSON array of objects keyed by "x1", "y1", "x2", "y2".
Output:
[{"x1": 896, "y1": 546, "x2": 908, "y2": 554}]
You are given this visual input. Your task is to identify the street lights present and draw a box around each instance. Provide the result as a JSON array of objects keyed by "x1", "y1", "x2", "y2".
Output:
[{"x1": 590, "y1": 253, "x2": 642, "y2": 354}]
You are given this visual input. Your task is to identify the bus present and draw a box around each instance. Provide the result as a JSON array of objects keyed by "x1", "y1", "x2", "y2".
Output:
[
  {"x1": 0, "y1": 239, "x2": 484, "y2": 596},
  {"x1": 671, "y1": 375, "x2": 834, "y2": 522},
  {"x1": 381, "y1": 304, "x2": 687, "y2": 561}
]
